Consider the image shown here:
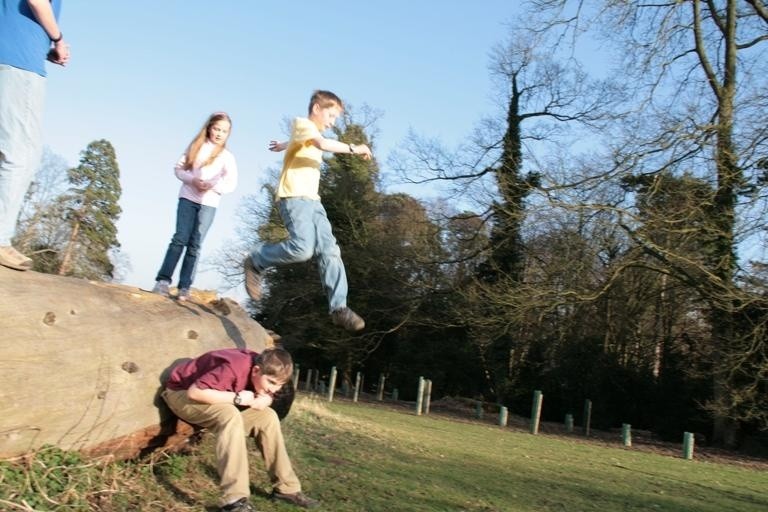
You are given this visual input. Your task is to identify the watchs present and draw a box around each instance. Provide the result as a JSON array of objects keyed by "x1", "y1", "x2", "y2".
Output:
[{"x1": 233, "y1": 391, "x2": 242, "y2": 406}]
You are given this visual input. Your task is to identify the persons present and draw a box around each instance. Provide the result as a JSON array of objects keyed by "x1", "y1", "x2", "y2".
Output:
[
  {"x1": 0, "y1": 1, "x2": 73, "y2": 272},
  {"x1": 243, "y1": 91, "x2": 374, "y2": 332},
  {"x1": 159, "y1": 348, "x2": 320, "y2": 511},
  {"x1": 151, "y1": 112, "x2": 234, "y2": 302}
]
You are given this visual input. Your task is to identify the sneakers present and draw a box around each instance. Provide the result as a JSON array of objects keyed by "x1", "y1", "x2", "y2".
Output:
[
  {"x1": 152, "y1": 279, "x2": 171, "y2": 299},
  {"x1": 176, "y1": 288, "x2": 191, "y2": 302},
  {"x1": 221, "y1": 494, "x2": 257, "y2": 512},
  {"x1": 270, "y1": 489, "x2": 323, "y2": 508},
  {"x1": 243, "y1": 256, "x2": 264, "y2": 305},
  {"x1": 328, "y1": 307, "x2": 365, "y2": 331},
  {"x1": 0, "y1": 246, "x2": 35, "y2": 271}
]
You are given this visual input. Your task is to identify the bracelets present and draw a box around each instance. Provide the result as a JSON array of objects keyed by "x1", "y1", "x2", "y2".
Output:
[
  {"x1": 50, "y1": 33, "x2": 64, "y2": 44},
  {"x1": 346, "y1": 141, "x2": 356, "y2": 155}
]
